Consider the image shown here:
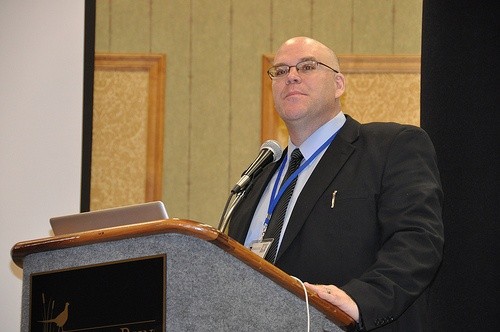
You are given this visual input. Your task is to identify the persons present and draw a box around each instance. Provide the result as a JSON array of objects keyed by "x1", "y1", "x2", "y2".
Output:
[{"x1": 228, "y1": 37, "x2": 445, "y2": 332}]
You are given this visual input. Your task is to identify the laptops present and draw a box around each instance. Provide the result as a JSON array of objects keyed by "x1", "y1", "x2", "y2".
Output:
[{"x1": 49, "y1": 201, "x2": 168, "y2": 236}]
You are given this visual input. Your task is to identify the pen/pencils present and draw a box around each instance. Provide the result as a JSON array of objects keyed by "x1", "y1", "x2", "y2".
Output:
[{"x1": 331, "y1": 189, "x2": 338, "y2": 210}]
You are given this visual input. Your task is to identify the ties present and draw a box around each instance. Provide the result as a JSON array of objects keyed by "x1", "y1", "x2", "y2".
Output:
[{"x1": 263, "y1": 148, "x2": 304, "y2": 265}]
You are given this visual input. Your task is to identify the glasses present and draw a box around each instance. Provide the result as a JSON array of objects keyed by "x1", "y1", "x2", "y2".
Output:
[{"x1": 266, "y1": 60, "x2": 339, "y2": 80}]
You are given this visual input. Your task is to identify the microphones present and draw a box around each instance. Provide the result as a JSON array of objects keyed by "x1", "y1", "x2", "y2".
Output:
[{"x1": 231, "y1": 140, "x2": 281, "y2": 194}]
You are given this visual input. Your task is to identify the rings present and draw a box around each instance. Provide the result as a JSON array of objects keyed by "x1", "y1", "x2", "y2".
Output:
[{"x1": 328, "y1": 290, "x2": 331, "y2": 294}]
